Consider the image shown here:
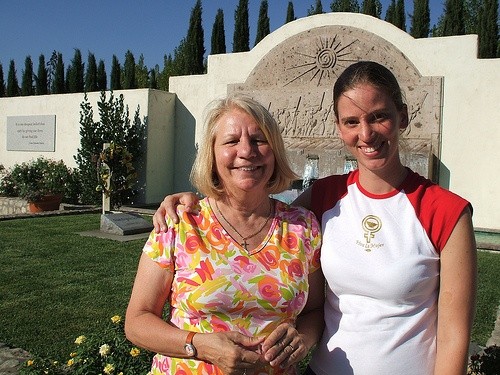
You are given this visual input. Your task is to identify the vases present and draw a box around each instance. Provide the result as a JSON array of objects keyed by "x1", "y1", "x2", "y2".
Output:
[{"x1": 30, "y1": 195, "x2": 62, "y2": 213}]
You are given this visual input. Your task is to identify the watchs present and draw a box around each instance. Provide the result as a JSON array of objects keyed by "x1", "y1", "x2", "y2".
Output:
[{"x1": 183, "y1": 332, "x2": 199, "y2": 360}]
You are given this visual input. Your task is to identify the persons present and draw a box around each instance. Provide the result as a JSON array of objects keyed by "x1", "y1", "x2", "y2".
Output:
[
  {"x1": 124, "y1": 96, "x2": 324, "y2": 375},
  {"x1": 153, "y1": 62, "x2": 477, "y2": 375}
]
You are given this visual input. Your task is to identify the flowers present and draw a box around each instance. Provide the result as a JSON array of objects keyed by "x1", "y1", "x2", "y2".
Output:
[{"x1": 11, "y1": 155, "x2": 73, "y2": 204}]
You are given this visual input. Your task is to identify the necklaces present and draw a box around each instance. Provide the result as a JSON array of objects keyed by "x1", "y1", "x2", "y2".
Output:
[{"x1": 215, "y1": 198, "x2": 272, "y2": 249}]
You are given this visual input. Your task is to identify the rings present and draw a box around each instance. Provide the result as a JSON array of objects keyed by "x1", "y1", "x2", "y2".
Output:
[{"x1": 288, "y1": 344, "x2": 294, "y2": 350}]
